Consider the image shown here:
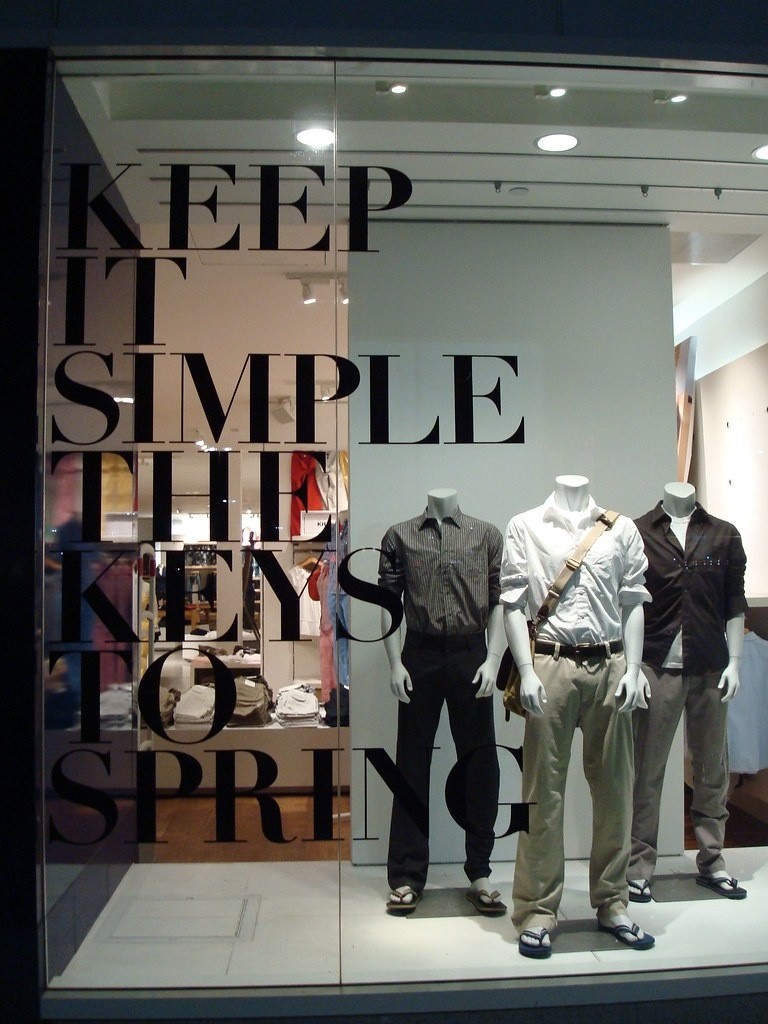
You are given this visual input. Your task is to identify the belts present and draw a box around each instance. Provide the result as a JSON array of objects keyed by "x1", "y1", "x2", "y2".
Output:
[{"x1": 534, "y1": 639, "x2": 624, "y2": 662}]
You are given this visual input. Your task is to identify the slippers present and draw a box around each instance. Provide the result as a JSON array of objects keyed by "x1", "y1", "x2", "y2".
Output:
[
  {"x1": 696, "y1": 876, "x2": 747, "y2": 900},
  {"x1": 598, "y1": 917, "x2": 655, "y2": 950},
  {"x1": 463, "y1": 889, "x2": 507, "y2": 912},
  {"x1": 519, "y1": 929, "x2": 552, "y2": 957},
  {"x1": 625, "y1": 879, "x2": 651, "y2": 902},
  {"x1": 386, "y1": 889, "x2": 423, "y2": 911}
]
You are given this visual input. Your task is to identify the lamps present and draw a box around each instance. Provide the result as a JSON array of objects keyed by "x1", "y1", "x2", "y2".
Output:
[
  {"x1": 376, "y1": 80, "x2": 406, "y2": 95},
  {"x1": 534, "y1": 83, "x2": 568, "y2": 99},
  {"x1": 652, "y1": 89, "x2": 688, "y2": 104},
  {"x1": 302, "y1": 283, "x2": 317, "y2": 306},
  {"x1": 337, "y1": 281, "x2": 348, "y2": 305}
]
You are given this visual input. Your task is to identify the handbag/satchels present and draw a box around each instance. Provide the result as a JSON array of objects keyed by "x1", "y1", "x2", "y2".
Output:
[{"x1": 496, "y1": 621, "x2": 538, "y2": 718}]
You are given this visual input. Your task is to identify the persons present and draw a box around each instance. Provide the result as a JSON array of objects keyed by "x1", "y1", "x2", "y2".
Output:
[
  {"x1": 499, "y1": 475, "x2": 656, "y2": 954},
  {"x1": 135, "y1": 556, "x2": 168, "y2": 674},
  {"x1": 378, "y1": 487, "x2": 509, "y2": 911},
  {"x1": 626, "y1": 482, "x2": 750, "y2": 901},
  {"x1": 243, "y1": 556, "x2": 255, "y2": 630}
]
